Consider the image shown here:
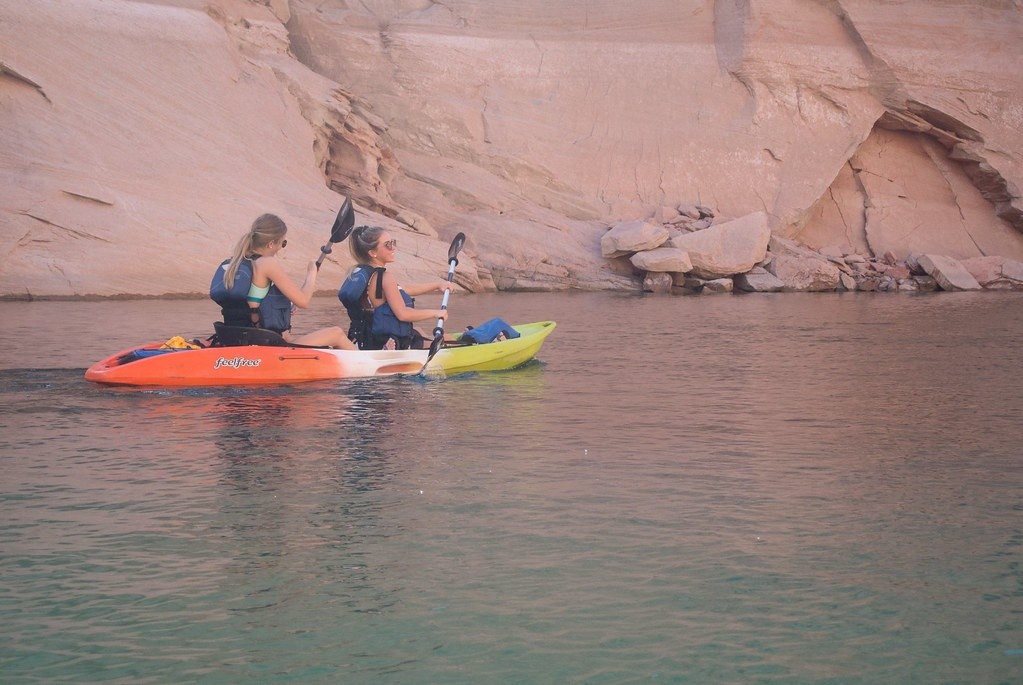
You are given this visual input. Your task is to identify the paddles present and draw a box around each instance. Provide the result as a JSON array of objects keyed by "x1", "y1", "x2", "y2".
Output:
[
  {"x1": 289, "y1": 194, "x2": 356, "y2": 315},
  {"x1": 416, "y1": 231, "x2": 467, "y2": 376}
]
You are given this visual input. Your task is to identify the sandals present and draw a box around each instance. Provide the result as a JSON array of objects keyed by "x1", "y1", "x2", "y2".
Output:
[{"x1": 381, "y1": 335, "x2": 400, "y2": 350}]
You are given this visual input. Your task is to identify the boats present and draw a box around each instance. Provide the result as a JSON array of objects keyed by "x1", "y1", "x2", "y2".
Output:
[{"x1": 86, "y1": 317, "x2": 557, "y2": 387}]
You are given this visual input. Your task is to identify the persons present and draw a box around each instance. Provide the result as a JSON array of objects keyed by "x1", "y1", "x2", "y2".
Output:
[
  {"x1": 223, "y1": 213, "x2": 400, "y2": 350},
  {"x1": 349, "y1": 225, "x2": 507, "y2": 350}
]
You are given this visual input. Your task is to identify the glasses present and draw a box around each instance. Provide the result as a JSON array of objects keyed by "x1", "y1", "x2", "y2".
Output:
[
  {"x1": 375, "y1": 240, "x2": 396, "y2": 250},
  {"x1": 279, "y1": 239, "x2": 287, "y2": 247}
]
